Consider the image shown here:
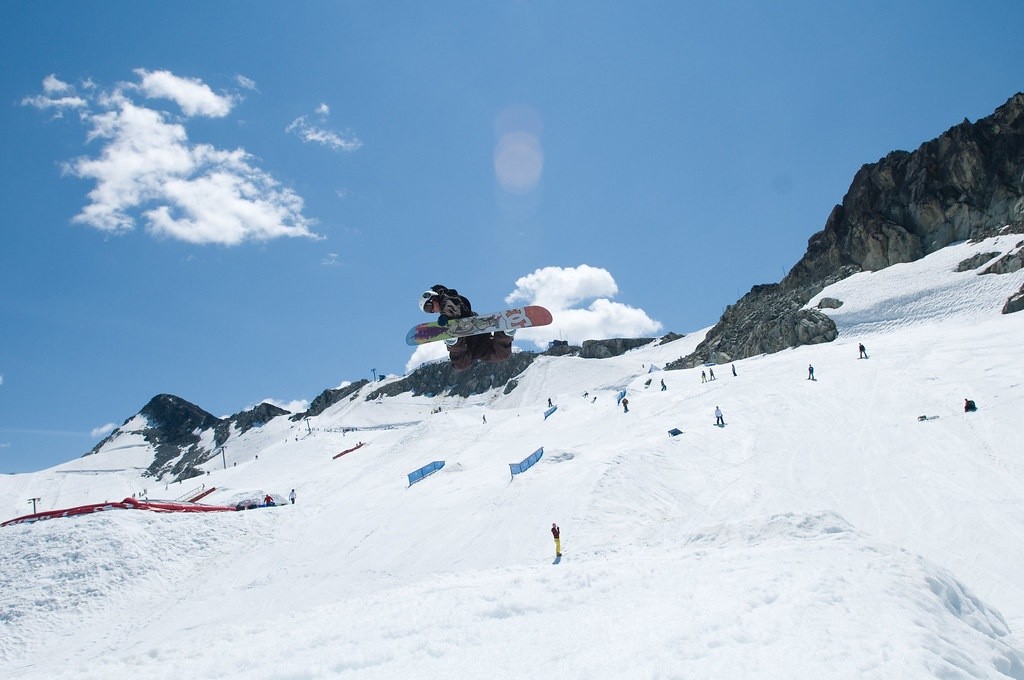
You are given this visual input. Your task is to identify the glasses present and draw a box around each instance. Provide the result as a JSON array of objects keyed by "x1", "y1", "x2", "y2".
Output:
[{"x1": 424, "y1": 299, "x2": 435, "y2": 313}]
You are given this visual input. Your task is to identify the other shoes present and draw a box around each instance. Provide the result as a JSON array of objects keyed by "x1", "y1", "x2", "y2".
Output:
[
  {"x1": 504, "y1": 329, "x2": 516, "y2": 336},
  {"x1": 445, "y1": 338, "x2": 458, "y2": 346}
]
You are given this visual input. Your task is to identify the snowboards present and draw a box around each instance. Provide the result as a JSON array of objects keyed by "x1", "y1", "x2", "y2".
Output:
[{"x1": 404, "y1": 305, "x2": 553, "y2": 348}]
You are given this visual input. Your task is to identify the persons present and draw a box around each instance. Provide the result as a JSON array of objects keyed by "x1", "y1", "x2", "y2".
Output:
[
  {"x1": 431, "y1": 406, "x2": 442, "y2": 414},
  {"x1": 701, "y1": 371, "x2": 707, "y2": 383},
  {"x1": 661, "y1": 379, "x2": 666, "y2": 391},
  {"x1": 548, "y1": 398, "x2": 553, "y2": 407},
  {"x1": 419, "y1": 284, "x2": 516, "y2": 369},
  {"x1": 551, "y1": 523, "x2": 562, "y2": 556},
  {"x1": 715, "y1": 406, "x2": 724, "y2": 425},
  {"x1": 732, "y1": 364, "x2": 737, "y2": 376},
  {"x1": 483, "y1": 415, "x2": 487, "y2": 424},
  {"x1": 709, "y1": 368, "x2": 715, "y2": 380},
  {"x1": 289, "y1": 489, "x2": 296, "y2": 504},
  {"x1": 965, "y1": 399, "x2": 975, "y2": 411},
  {"x1": 808, "y1": 364, "x2": 814, "y2": 380},
  {"x1": 264, "y1": 495, "x2": 273, "y2": 506},
  {"x1": 582, "y1": 391, "x2": 589, "y2": 398},
  {"x1": 622, "y1": 398, "x2": 628, "y2": 411},
  {"x1": 859, "y1": 343, "x2": 867, "y2": 358},
  {"x1": 591, "y1": 396, "x2": 596, "y2": 403}
]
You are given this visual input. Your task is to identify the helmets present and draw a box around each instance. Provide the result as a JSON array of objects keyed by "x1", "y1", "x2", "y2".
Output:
[{"x1": 419, "y1": 291, "x2": 440, "y2": 314}]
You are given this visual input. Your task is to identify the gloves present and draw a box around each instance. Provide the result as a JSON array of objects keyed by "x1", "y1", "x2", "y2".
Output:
[{"x1": 438, "y1": 315, "x2": 449, "y2": 326}]
